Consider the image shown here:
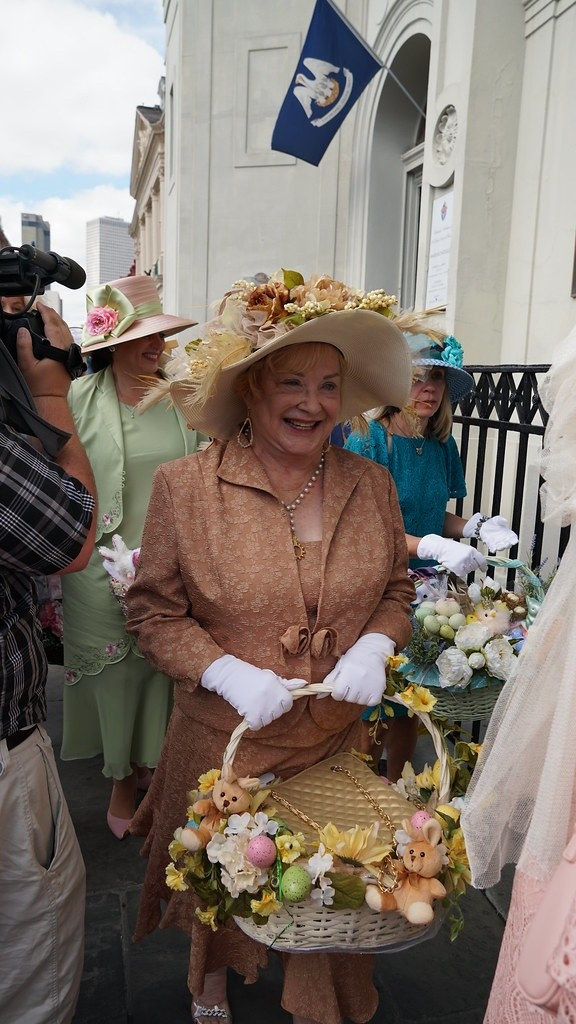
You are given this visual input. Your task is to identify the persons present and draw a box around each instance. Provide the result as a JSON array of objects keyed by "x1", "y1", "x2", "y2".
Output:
[
  {"x1": 459, "y1": 323, "x2": 576, "y2": 1023},
  {"x1": 125, "y1": 267, "x2": 418, "y2": 1024},
  {"x1": 342, "y1": 331, "x2": 518, "y2": 785},
  {"x1": 0, "y1": 301, "x2": 98, "y2": 1024},
  {"x1": 0, "y1": 296, "x2": 47, "y2": 314},
  {"x1": 330, "y1": 407, "x2": 380, "y2": 447},
  {"x1": 62, "y1": 275, "x2": 212, "y2": 842}
]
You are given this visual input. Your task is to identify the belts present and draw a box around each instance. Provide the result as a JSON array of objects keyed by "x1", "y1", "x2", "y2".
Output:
[{"x1": 6, "y1": 725, "x2": 37, "y2": 750}]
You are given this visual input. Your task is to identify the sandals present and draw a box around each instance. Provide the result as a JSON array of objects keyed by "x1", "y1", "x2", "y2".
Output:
[{"x1": 191, "y1": 974, "x2": 231, "y2": 1024}]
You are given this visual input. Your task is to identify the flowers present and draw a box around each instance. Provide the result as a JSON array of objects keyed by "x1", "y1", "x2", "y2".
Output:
[
  {"x1": 166, "y1": 655, "x2": 482, "y2": 935},
  {"x1": 82, "y1": 286, "x2": 138, "y2": 347},
  {"x1": 187, "y1": 269, "x2": 448, "y2": 379},
  {"x1": 400, "y1": 533, "x2": 562, "y2": 689},
  {"x1": 439, "y1": 336, "x2": 465, "y2": 368},
  {"x1": 38, "y1": 597, "x2": 64, "y2": 642}
]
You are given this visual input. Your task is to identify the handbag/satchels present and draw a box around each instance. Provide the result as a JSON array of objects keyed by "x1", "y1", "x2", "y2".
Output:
[{"x1": 259, "y1": 753, "x2": 421, "y2": 856}]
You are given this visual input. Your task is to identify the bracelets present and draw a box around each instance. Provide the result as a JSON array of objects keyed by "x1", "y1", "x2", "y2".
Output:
[{"x1": 473, "y1": 516, "x2": 491, "y2": 541}]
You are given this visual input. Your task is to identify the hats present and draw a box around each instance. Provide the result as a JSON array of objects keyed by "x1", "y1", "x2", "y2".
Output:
[
  {"x1": 139, "y1": 268, "x2": 448, "y2": 440},
  {"x1": 404, "y1": 331, "x2": 475, "y2": 404},
  {"x1": 80, "y1": 276, "x2": 199, "y2": 356}
]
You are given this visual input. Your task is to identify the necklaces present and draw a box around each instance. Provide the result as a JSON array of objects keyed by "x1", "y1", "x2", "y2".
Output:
[
  {"x1": 394, "y1": 419, "x2": 426, "y2": 455},
  {"x1": 281, "y1": 450, "x2": 324, "y2": 560},
  {"x1": 116, "y1": 382, "x2": 134, "y2": 419}
]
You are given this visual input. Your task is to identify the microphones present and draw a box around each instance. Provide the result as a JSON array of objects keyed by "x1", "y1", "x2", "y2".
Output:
[{"x1": 20, "y1": 244, "x2": 86, "y2": 290}]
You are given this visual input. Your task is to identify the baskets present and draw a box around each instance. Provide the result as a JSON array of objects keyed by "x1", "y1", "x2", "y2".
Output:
[
  {"x1": 410, "y1": 556, "x2": 545, "y2": 721},
  {"x1": 221, "y1": 682, "x2": 451, "y2": 952}
]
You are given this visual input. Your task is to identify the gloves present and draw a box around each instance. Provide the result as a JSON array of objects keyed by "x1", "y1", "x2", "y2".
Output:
[
  {"x1": 201, "y1": 654, "x2": 308, "y2": 731},
  {"x1": 417, "y1": 534, "x2": 488, "y2": 578},
  {"x1": 463, "y1": 512, "x2": 519, "y2": 553},
  {"x1": 315, "y1": 633, "x2": 397, "y2": 706}
]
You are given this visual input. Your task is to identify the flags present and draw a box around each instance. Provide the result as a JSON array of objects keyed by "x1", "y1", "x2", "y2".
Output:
[{"x1": 271, "y1": 0, "x2": 382, "y2": 168}]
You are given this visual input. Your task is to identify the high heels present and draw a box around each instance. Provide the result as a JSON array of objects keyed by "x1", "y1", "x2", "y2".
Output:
[
  {"x1": 138, "y1": 769, "x2": 152, "y2": 790},
  {"x1": 107, "y1": 778, "x2": 136, "y2": 840}
]
